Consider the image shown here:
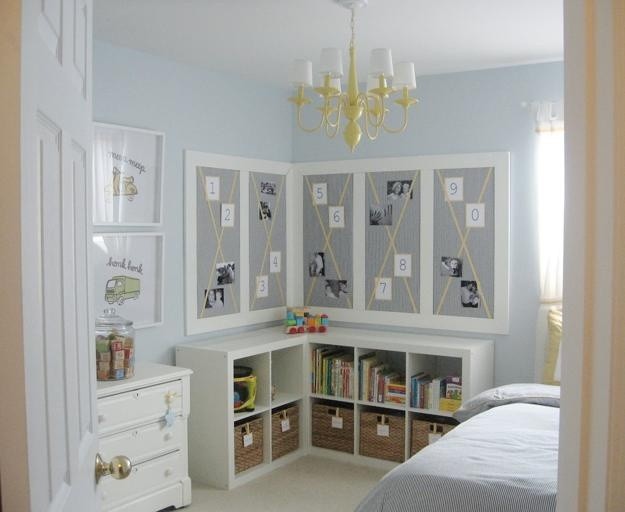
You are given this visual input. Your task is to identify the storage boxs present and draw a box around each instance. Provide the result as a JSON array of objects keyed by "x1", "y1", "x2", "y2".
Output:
[
  {"x1": 359, "y1": 408, "x2": 405, "y2": 463},
  {"x1": 312, "y1": 402, "x2": 354, "y2": 454},
  {"x1": 272, "y1": 403, "x2": 299, "y2": 461},
  {"x1": 410, "y1": 415, "x2": 460, "y2": 457},
  {"x1": 234, "y1": 417, "x2": 263, "y2": 474}
]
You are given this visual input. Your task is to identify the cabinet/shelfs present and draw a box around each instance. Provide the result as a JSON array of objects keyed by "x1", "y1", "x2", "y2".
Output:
[
  {"x1": 308, "y1": 325, "x2": 495, "y2": 470},
  {"x1": 97, "y1": 362, "x2": 194, "y2": 511},
  {"x1": 174, "y1": 324, "x2": 308, "y2": 491}
]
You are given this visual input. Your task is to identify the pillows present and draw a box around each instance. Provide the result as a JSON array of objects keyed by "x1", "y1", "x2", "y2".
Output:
[{"x1": 545, "y1": 307, "x2": 563, "y2": 386}]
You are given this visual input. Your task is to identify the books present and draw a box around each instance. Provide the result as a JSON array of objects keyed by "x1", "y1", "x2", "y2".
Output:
[
  {"x1": 312, "y1": 347, "x2": 354, "y2": 399},
  {"x1": 358, "y1": 351, "x2": 406, "y2": 406},
  {"x1": 410, "y1": 371, "x2": 461, "y2": 410}
]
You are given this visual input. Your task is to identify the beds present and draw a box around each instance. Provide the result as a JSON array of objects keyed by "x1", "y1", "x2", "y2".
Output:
[{"x1": 353, "y1": 303, "x2": 563, "y2": 512}]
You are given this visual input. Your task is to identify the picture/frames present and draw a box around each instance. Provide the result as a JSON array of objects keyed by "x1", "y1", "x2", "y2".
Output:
[
  {"x1": 183, "y1": 149, "x2": 294, "y2": 336},
  {"x1": 92, "y1": 231, "x2": 166, "y2": 331},
  {"x1": 92, "y1": 121, "x2": 167, "y2": 227},
  {"x1": 294, "y1": 151, "x2": 511, "y2": 335}
]
